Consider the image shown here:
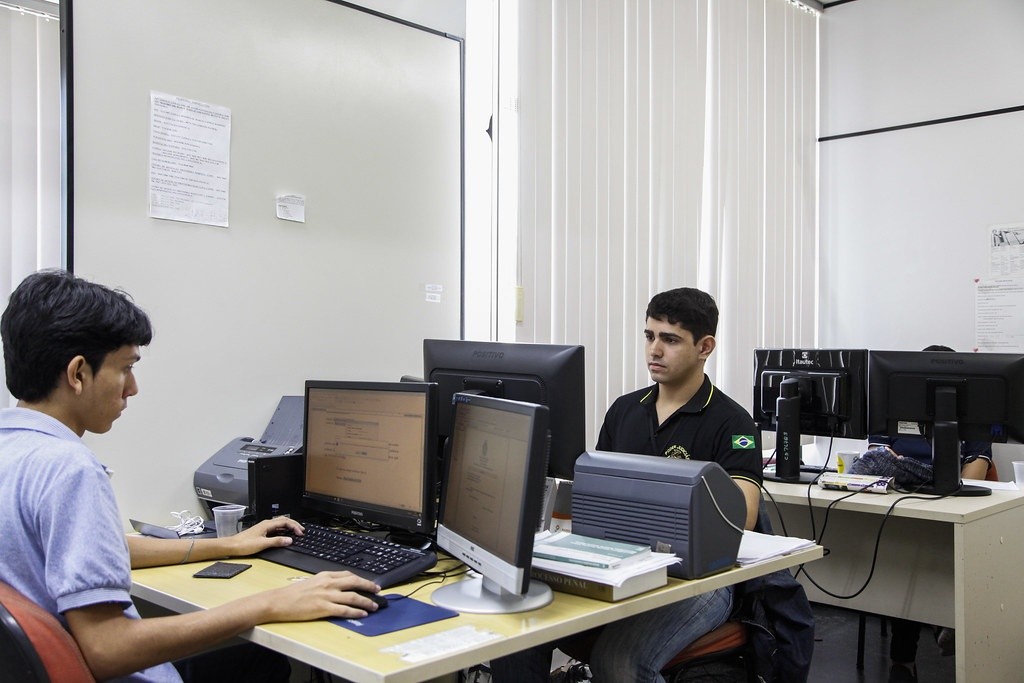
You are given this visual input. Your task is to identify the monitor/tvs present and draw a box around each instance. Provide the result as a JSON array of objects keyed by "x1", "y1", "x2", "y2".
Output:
[
  {"x1": 867, "y1": 350, "x2": 1024, "y2": 497},
  {"x1": 301, "y1": 337, "x2": 585, "y2": 614},
  {"x1": 752, "y1": 349, "x2": 868, "y2": 484}
]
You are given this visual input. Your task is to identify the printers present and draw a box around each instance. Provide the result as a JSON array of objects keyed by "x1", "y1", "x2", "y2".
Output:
[{"x1": 192, "y1": 395, "x2": 306, "y2": 529}]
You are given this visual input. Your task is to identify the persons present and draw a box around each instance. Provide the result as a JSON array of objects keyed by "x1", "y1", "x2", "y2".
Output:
[
  {"x1": 489, "y1": 288, "x2": 764, "y2": 683},
  {"x1": 868, "y1": 345, "x2": 992, "y2": 683},
  {"x1": 0, "y1": 266, "x2": 380, "y2": 683}
]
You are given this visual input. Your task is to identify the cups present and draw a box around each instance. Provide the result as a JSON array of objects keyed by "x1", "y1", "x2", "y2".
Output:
[
  {"x1": 212, "y1": 505, "x2": 247, "y2": 538},
  {"x1": 837, "y1": 451, "x2": 860, "y2": 474},
  {"x1": 1012, "y1": 461, "x2": 1024, "y2": 490}
]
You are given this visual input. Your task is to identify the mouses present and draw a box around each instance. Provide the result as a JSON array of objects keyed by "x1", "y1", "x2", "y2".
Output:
[{"x1": 337, "y1": 589, "x2": 388, "y2": 611}]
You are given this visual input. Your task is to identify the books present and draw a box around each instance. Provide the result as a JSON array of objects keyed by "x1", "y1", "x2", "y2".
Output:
[{"x1": 531, "y1": 531, "x2": 668, "y2": 603}]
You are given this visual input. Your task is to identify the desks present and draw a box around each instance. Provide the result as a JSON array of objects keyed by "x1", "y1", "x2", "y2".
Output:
[
  {"x1": 130, "y1": 544, "x2": 824, "y2": 683},
  {"x1": 762, "y1": 480, "x2": 1024, "y2": 683}
]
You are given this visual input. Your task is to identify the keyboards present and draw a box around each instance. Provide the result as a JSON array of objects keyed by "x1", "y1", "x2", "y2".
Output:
[
  {"x1": 799, "y1": 464, "x2": 838, "y2": 474},
  {"x1": 260, "y1": 521, "x2": 439, "y2": 591}
]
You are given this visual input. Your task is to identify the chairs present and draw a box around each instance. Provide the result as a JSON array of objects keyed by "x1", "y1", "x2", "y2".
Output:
[
  {"x1": 0, "y1": 580, "x2": 97, "y2": 683},
  {"x1": 660, "y1": 622, "x2": 749, "y2": 683}
]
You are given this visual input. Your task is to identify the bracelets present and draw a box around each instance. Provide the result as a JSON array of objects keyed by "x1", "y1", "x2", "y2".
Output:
[{"x1": 181, "y1": 537, "x2": 194, "y2": 564}]
